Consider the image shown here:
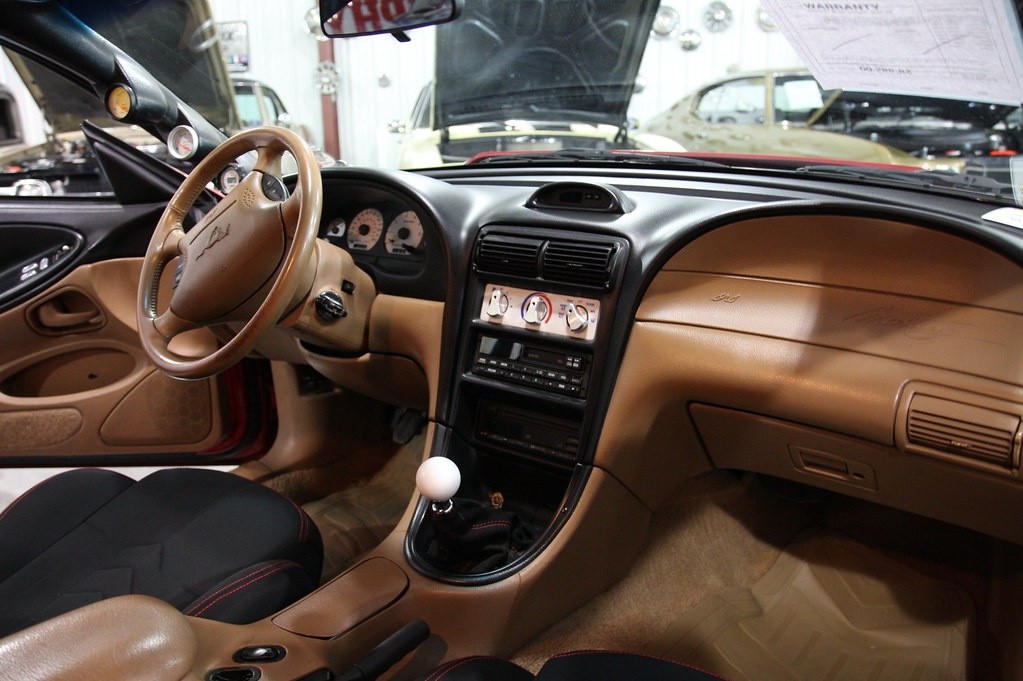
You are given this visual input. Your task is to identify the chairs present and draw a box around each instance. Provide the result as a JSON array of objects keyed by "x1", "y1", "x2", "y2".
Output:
[{"x1": 0, "y1": 465, "x2": 328, "y2": 640}]
